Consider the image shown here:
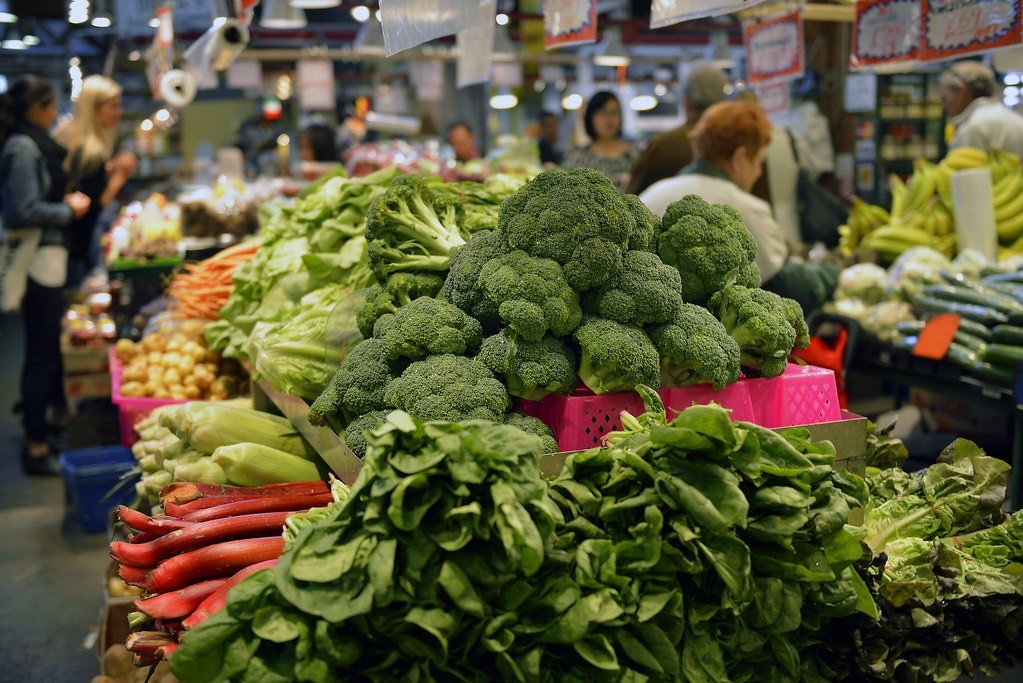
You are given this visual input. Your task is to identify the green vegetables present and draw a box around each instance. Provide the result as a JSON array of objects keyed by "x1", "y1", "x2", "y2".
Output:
[
  {"x1": 205, "y1": 161, "x2": 528, "y2": 403},
  {"x1": 167, "y1": 383, "x2": 1023, "y2": 683}
]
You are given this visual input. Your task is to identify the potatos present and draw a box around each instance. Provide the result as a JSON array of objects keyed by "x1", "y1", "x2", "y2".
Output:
[{"x1": 115, "y1": 317, "x2": 249, "y2": 402}]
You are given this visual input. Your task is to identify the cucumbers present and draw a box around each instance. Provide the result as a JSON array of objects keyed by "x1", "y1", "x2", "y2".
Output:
[{"x1": 892, "y1": 263, "x2": 1023, "y2": 381}]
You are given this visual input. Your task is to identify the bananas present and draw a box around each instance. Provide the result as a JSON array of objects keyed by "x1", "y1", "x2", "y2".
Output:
[{"x1": 837, "y1": 147, "x2": 1023, "y2": 259}]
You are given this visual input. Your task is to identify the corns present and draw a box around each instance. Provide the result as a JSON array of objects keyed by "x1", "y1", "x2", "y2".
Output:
[{"x1": 133, "y1": 401, "x2": 331, "y2": 499}]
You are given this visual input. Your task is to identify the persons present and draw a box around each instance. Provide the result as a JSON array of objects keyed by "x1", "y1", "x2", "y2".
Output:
[
  {"x1": 538, "y1": 113, "x2": 563, "y2": 165},
  {"x1": 448, "y1": 123, "x2": 485, "y2": 164},
  {"x1": 51, "y1": 73, "x2": 138, "y2": 421},
  {"x1": 297, "y1": 122, "x2": 345, "y2": 165},
  {"x1": 638, "y1": 102, "x2": 839, "y2": 308},
  {"x1": 624, "y1": 64, "x2": 770, "y2": 198},
  {"x1": 938, "y1": 61, "x2": 1023, "y2": 160},
  {"x1": 561, "y1": 91, "x2": 647, "y2": 194},
  {"x1": 0, "y1": 74, "x2": 91, "y2": 477}
]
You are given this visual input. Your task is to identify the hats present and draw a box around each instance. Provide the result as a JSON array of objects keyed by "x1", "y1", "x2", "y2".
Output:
[{"x1": 687, "y1": 69, "x2": 727, "y2": 106}]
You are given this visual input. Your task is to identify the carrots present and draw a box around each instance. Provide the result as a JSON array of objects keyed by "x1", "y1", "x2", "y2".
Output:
[{"x1": 168, "y1": 239, "x2": 261, "y2": 322}]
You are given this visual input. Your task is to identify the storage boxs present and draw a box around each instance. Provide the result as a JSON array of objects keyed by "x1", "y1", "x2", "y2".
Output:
[
  {"x1": 60, "y1": 446, "x2": 139, "y2": 534},
  {"x1": 105, "y1": 346, "x2": 209, "y2": 448}
]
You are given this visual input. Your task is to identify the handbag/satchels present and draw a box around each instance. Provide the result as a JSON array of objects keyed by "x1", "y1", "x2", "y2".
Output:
[
  {"x1": 797, "y1": 172, "x2": 855, "y2": 248},
  {"x1": 0, "y1": 228, "x2": 46, "y2": 311}
]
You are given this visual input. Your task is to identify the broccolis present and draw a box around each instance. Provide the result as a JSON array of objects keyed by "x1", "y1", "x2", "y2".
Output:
[
  {"x1": 822, "y1": 246, "x2": 1023, "y2": 343},
  {"x1": 309, "y1": 169, "x2": 809, "y2": 455}
]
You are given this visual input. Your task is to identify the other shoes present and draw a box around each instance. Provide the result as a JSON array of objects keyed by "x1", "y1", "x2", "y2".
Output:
[{"x1": 24, "y1": 449, "x2": 61, "y2": 474}]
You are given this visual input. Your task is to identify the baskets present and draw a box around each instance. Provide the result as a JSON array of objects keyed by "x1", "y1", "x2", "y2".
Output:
[
  {"x1": 518, "y1": 361, "x2": 841, "y2": 454},
  {"x1": 107, "y1": 348, "x2": 207, "y2": 445}
]
